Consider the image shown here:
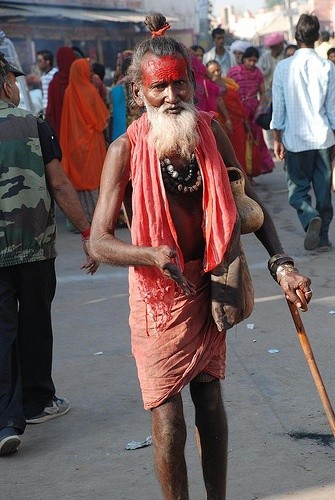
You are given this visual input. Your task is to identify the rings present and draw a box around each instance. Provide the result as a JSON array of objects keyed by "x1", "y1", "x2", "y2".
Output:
[{"x1": 305, "y1": 289, "x2": 313, "y2": 294}]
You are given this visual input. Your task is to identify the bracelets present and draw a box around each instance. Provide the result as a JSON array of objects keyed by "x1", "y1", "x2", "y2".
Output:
[
  {"x1": 82, "y1": 226, "x2": 94, "y2": 240},
  {"x1": 268, "y1": 254, "x2": 297, "y2": 285}
]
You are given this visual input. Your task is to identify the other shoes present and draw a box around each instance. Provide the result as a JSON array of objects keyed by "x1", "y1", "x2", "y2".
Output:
[
  {"x1": 319, "y1": 241, "x2": 333, "y2": 251},
  {"x1": 304, "y1": 217, "x2": 322, "y2": 250}
]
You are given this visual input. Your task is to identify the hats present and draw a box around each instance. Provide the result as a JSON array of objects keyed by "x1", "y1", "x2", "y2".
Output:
[
  {"x1": 264, "y1": 33, "x2": 284, "y2": 46},
  {"x1": 230, "y1": 40, "x2": 252, "y2": 52},
  {"x1": 0, "y1": 52, "x2": 28, "y2": 77}
]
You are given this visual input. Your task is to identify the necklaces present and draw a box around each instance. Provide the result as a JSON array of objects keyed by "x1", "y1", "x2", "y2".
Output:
[
  {"x1": 167, "y1": 171, "x2": 202, "y2": 193},
  {"x1": 163, "y1": 153, "x2": 199, "y2": 185},
  {"x1": 160, "y1": 160, "x2": 203, "y2": 195}
]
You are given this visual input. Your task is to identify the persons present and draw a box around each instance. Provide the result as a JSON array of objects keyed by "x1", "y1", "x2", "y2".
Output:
[
  {"x1": 36, "y1": 49, "x2": 61, "y2": 121},
  {"x1": 60, "y1": 57, "x2": 110, "y2": 234},
  {"x1": 203, "y1": 28, "x2": 238, "y2": 77},
  {"x1": 270, "y1": 14, "x2": 335, "y2": 252},
  {"x1": 73, "y1": 45, "x2": 148, "y2": 149},
  {"x1": 315, "y1": 31, "x2": 335, "y2": 62},
  {"x1": 86, "y1": 12, "x2": 313, "y2": 500},
  {"x1": 0, "y1": 51, "x2": 100, "y2": 458},
  {"x1": 188, "y1": 41, "x2": 299, "y2": 186},
  {"x1": 258, "y1": 31, "x2": 289, "y2": 114},
  {"x1": 46, "y1": 46, "x2": 77, "y2": 141}
]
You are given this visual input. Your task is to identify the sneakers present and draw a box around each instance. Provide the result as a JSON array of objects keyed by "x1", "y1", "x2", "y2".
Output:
[
  {"x1": 0, "y1": 427, "x2": 20, "y2": 455},
  {"x1": 25, "y1": 395, "x2": 70, "y2": 423}
]
